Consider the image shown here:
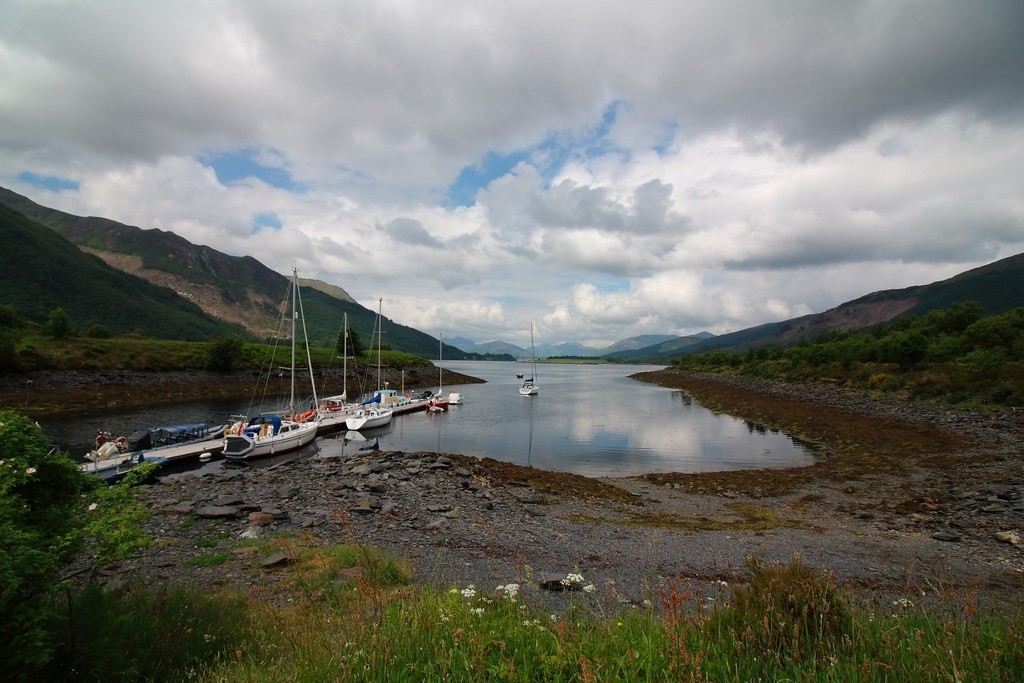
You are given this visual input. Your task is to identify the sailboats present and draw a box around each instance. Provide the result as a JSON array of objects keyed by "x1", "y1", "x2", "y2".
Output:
[
  {"x1": 426, "y1": 332, "x2": 449, "y2": 412},
  {"x1": 220, "y1": 258, "x2": 318, "y2": 460},
  {"x1": 308, "y1": 296, "x2": 393, "y2": 432},
  {"x1": 519, "y1": 322, "x2": 539, "y2": 395}
]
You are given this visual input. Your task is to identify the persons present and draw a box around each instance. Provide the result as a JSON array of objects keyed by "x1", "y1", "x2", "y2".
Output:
[
  {"x1": 406, "y1": 391, "x2": 410, "y2": 398},
  {"x1": 429, "y1": 401, "x2": 434, "y2": 406}
]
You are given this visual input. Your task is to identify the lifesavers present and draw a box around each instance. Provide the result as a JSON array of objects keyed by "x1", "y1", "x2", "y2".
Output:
[
  {"x1": 295, "y1": 414, "x2": 301, "y2": 423},
  {"x1": 405, "y1": 392, "x2": 411, "y2": 398},
  {"x1": 115, "y1": 436, "x2": 128, "y2": 449},
  {"x1": 300, "y1": 414, "x2": 305, "y2": 421},
  {"x1": 224, "y1": 429, "x2": 232, "y2": 435},
  {"x1": 95, "y1": 435, "x2": 109, "y2": 447},
  {"x1": 327, "y1": 405, "x2": 332, "y2": 413}
]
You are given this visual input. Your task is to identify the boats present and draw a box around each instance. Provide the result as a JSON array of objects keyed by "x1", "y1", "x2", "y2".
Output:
[
  {"x1": 1, "y1": 423, "x2": 229, "y2": 480},
  {"x1": 517, "y1": 374, "x2": 523, "y2": 378}
]
